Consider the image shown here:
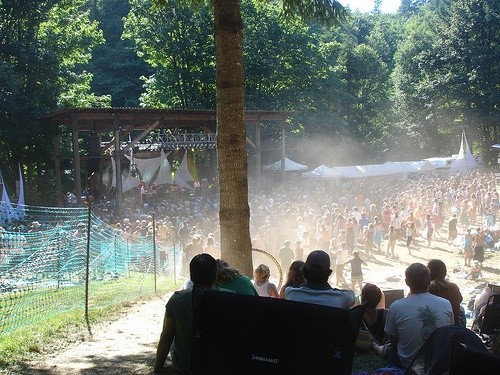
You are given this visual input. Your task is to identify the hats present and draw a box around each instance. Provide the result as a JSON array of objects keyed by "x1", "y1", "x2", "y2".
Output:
[
  {"x1": 304, "y1": 250, "x2": 331, "y2": 273},
  {"x1": 31, "y1": 222, "x2": 41, "y2": 227}
]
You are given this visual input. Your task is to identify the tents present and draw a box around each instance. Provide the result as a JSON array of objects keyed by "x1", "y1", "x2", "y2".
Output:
[
  {"x1": 302, "y1": 164, "x2": 342, "y2": 179},
  {"x1": 261, "y1": 157, "x2": 308, "y2": 171}
]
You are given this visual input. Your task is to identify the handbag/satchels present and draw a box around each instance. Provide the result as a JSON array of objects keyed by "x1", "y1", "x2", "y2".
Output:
[{"x1": 356, "y1": 319, "x2": 373, "y2": 353}]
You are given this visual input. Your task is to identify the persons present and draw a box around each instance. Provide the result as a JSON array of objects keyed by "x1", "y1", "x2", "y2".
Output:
[
  {"x1": 216, "y1": 259, "x2": 260, "y2": 296},
  {"x1": 427, "y1": 258, "x2": 463, "y2": 326},
  {"x1": 250, "y1": 264, "x2": 279, "y2": 298},
  {"x1": 154, "y1": 253, "x2": 234, "y2": 375},
  {"x1": 361, "y1": 283, "x2": 389, "y2": 345},
  {"x1": 280, "y1": 260, "x2": 305, "y2": 299},
  {"x1": 373, "y1": 263, "x2": 455, "y2": 369},
  {"x1": 0, "y1": 170, "x2": 500, "y2": 290},
  {"x1": 285, "y1": 250, "x2": 355, "y2": 309}
]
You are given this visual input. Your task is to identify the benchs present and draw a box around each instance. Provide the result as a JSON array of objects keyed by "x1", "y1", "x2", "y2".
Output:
[{"x1": 188, "y1": 285, "x2": 364, "y2": 375}]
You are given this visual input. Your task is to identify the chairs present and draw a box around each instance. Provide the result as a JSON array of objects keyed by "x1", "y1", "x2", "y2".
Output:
[{"x1": 382, "y1": 286, "x2": 500, "y2": 375}]
